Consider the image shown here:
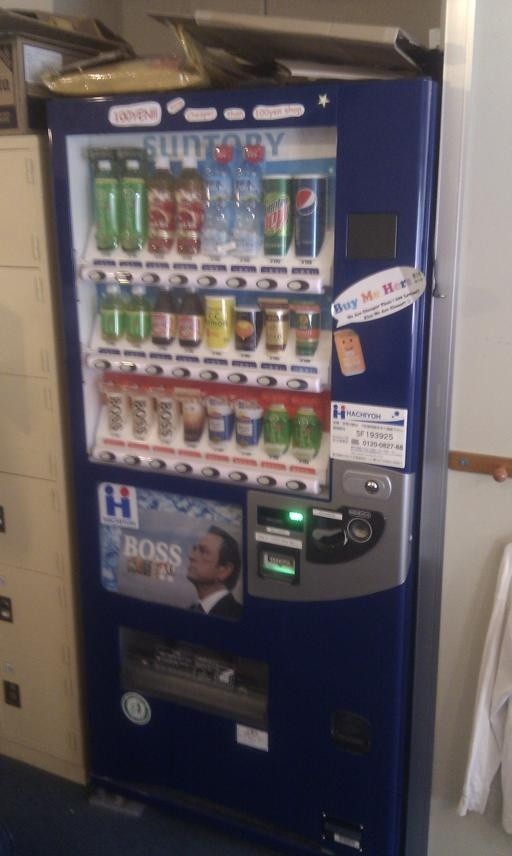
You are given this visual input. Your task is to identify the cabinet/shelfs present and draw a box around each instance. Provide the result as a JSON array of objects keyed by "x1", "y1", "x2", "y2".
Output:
[{"x1": 0, "y1": 132, "x2": 87, "y2": 789}]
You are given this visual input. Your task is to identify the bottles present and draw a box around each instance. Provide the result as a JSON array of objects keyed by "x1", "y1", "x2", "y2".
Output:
[{"x1": 93, "y1": 145, "x2": 265, "y2": 347}]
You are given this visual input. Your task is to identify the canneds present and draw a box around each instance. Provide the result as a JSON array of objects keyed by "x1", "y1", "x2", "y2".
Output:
[
  {"x1": 333, "y1": 328, "x2": 366, "y2": 376},
  {"x1": 265, "y1": 308, "x2": 289, "y2": 351},
  {"x1": 261, "y1": 174, "x2": 294, "y2": 256},
  {"x1": 234, "y1": 304, "x2": 263, "y2": 351},
  {"x1": 203, "y1": 295, "x2": 235, "y2": 350},
  {"x1": 294, "y1": 174, "x2": 326, "y2": 257},
  {"x1": 296, "y1": 310, "x2": 320, "y2": 356}
]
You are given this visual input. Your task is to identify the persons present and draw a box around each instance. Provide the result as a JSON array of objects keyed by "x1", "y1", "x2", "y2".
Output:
[{"x1": 186, "y1": 527, "x2": 242, "y2": 619}]
